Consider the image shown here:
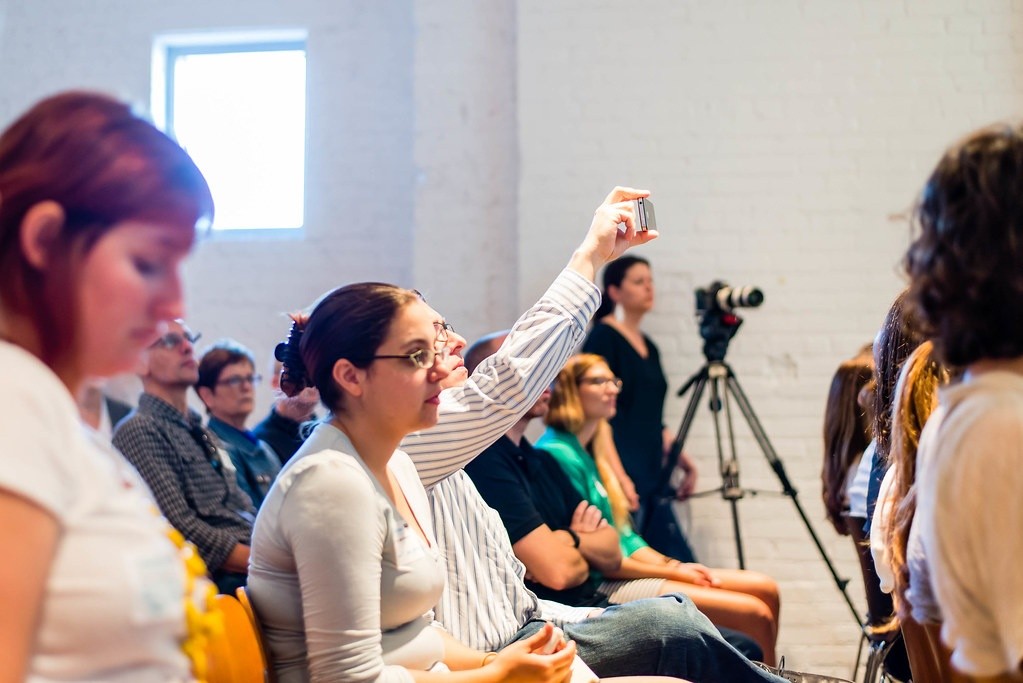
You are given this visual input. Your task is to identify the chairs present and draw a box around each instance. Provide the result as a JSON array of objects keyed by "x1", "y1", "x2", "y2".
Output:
[{"x1": 844, "y1": 516, "x2": 902, "y2": 683}]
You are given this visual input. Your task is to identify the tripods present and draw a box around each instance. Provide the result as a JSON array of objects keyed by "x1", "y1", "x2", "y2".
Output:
[{"x1": 658, "y1": 337, "x2": 873, "y2": 646}]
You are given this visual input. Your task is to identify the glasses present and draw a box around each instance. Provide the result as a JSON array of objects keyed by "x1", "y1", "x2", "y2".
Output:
[
  {"x1": 433, "y1": 322, "x2": 454, "y2": 342},
  {"x1": 578, "y1": 377, "x2": 623, "y2": 388},
  {"x1": 212, "y1": 373, "x2": 262, "y2": 388},
  {"x1": 148, "y1": 330, "x2": 197, "y2": 349},
  {"x1": 348, "y1": 348, "x2": 451, "y2": 370}
]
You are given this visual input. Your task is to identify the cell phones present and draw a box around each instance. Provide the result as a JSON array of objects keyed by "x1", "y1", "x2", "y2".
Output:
[{"x1": 637, "y1": 196, "x2": 657, "y2": 231}]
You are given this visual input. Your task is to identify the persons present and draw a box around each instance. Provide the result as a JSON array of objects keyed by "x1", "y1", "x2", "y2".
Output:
[{"x1": 0, "y1": 92, "x2": 1023, "y2": 683}]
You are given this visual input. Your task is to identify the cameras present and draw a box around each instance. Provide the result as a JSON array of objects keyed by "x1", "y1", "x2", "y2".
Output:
[{"x1": 696, "y1": 282, "x2": 764, "y2": 337}]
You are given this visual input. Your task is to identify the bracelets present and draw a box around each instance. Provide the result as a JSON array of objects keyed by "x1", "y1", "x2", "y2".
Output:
[
  {"x1": 481, "y1": 652, "x2": 497, "y2": 666},
  {"x1": 565, "y1": 528, "x2": 580, "y2": 548}
]
